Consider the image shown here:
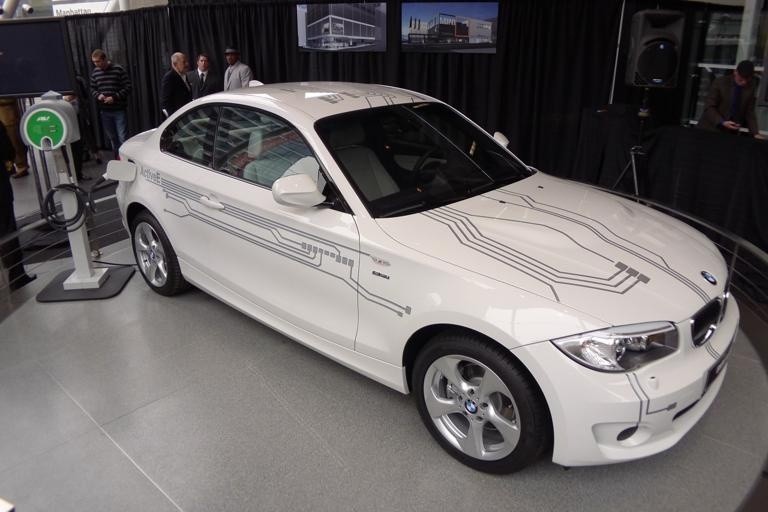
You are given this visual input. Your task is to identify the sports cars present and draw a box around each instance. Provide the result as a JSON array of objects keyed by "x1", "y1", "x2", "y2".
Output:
[{"x1": 96, "y1": 73, "x2": 746, "y2": 480}]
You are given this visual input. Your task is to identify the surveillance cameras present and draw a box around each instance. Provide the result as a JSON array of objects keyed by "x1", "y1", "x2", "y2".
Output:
[{"x1": 21, "y1": 4, "x2": 34, "y2": 15}]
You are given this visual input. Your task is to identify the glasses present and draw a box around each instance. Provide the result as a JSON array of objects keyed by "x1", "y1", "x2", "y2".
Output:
[{"x1": 224, "y1": 51, "x2": 238, "y2": 56}]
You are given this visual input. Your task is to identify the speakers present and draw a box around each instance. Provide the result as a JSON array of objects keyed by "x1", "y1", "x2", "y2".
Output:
[{"x1": 624, "y1": 7, "x2": 687, "y2": 90}]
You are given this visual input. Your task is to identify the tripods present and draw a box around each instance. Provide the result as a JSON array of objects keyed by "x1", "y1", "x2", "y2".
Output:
[{"x1": 612, "y1": 89, "x2": 657, "y2": 208}]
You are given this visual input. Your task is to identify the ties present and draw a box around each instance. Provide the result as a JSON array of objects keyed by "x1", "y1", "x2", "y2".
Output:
[
  {"x1": 200, "y1": 73, "x2": 204, "y2": 86},
  {"x1": 228, "y1": 70, "x2": 232, "y2": 82},
  {"x1": 185, "y1": 76, "x2": 192, "y2": 92}
]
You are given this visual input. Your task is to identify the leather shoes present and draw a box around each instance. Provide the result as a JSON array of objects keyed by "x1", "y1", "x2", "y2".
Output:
[{"x1": 13, "y1": 276, "x2": 35, "y2": 285}]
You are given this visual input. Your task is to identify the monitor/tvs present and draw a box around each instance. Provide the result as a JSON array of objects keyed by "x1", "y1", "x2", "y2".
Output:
[
  {"x1": 0, "y1": 16, "x2": 79, "y2": 100},
  {"x1": 291, "y1": 2, "x2": 392, "y2": 57},
  {"x1": 394, "y1": 1, "x2": 505, "y2": 59}
]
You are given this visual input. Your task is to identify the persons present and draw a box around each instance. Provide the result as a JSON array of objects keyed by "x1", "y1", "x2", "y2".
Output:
[
  {"x1": 186, "y1": 53, "x2": 224, "y2": 99},
  {"x1": 159, "y1": 52, "x2": 192, "y2": 123},
  {"x1": 89, "y1": 49, "x2": 131, "y2": 160},
  {"x1": 0, "y1": 120, "x2": 37, "y2": 290},
  {"x1": 697, "y1": 60, "x2": 768, "y2": 140},
  {"x1": 224, "y1": 47, "x2": 254, "y2": 91},
  {"x1": 61, "y1": 76, "x2": 92, "y2": 183}
]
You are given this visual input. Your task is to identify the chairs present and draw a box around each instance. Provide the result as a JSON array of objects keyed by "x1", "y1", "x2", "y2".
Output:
[
  {"x1": 243, "y1": 129, "x2": 295, "y2": 188},
  {"x1": 330, "y1": 120, "x2": 400, "y2": 202}
]
